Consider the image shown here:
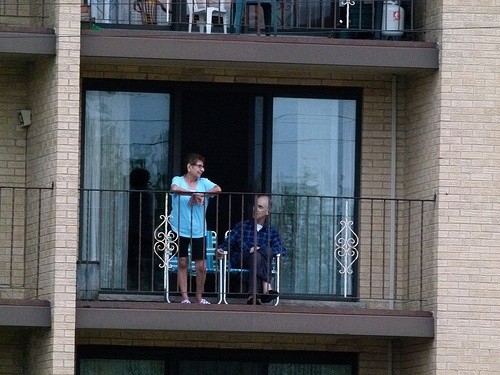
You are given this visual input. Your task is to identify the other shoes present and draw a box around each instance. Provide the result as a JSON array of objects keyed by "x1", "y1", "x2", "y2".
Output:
[
  {"x1": 197, "y1": 299, "x2": 212, "y2": 304},
  {"x1": 180, "y1": 300, "x2": 191, "y2": 304},
  {"x1": 246, "y1": 298, "x2": 261, "y2": 306},
  {"x1": 261, "y1": 290, "x2": 279, "y2": 303}
]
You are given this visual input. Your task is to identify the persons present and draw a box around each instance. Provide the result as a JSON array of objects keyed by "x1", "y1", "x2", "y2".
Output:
[
  {"x1": 217, "y1": 195, "x2": 285, "y2": 305},
  {"x1": 168, "y1": 153, "x2": 221, "y2": 304}
]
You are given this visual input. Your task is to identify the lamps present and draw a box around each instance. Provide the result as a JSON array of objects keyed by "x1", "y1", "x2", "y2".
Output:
[{"x1": 15, "y1": 109, "x2": 32, "y2": 127}]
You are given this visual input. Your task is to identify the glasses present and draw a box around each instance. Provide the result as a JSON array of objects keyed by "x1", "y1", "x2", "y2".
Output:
[{"x1": 191, "y1": 163, "x2": 205, "y2": 169}]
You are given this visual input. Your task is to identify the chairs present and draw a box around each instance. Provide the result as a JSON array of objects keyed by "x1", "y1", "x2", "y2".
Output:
[
  {"x1": 231, "y1": 0, "x2": 277, "y2": 36},
  {"x1": 133, "y1": 0, "x2": 176, "y2": 31},
  {"x1": 164, "y1": 230, "x2": 224, "y2": 304},
  {"x1": 222, "y1": 230, "x2": 281, "y2": 306},
  {"x1": 186, "y1": 0, "x2": 227, "y2": 34}
]
